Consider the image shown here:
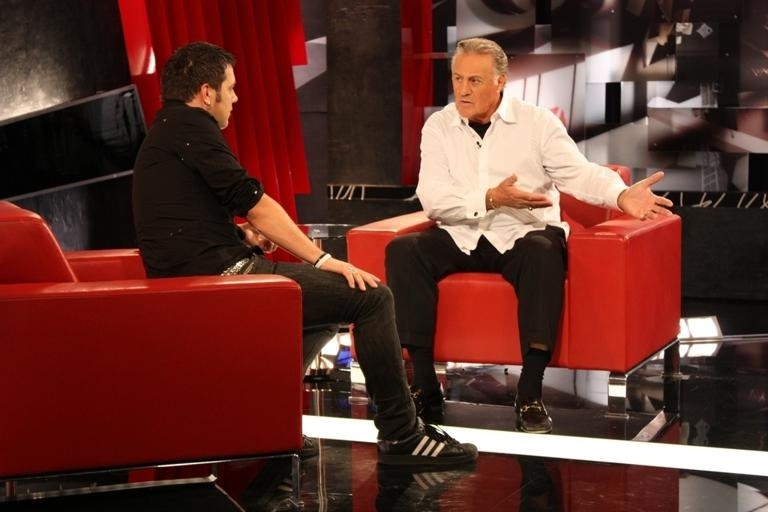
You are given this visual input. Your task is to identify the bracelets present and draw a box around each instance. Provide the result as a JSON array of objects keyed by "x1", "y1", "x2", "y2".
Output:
[
  {"x1": 490, "y1": 188, "x2": 498, "y2": 209},
  {"x1": 313, "y1": 253, "x2": 332, "y2": 269}
]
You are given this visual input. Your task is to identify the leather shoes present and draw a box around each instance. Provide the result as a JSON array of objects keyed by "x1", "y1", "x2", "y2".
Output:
[
  {"x1": 410, "y1": 386, "x2": 446, "y2": 416},
  {"x1": 514, "y1": 396, "x2": 553, "y2": 433}
]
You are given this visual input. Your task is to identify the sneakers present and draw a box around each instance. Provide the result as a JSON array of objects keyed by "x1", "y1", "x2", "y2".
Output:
[{"x1": 377, "y1": 418, "x2": 478, "y2": 469}]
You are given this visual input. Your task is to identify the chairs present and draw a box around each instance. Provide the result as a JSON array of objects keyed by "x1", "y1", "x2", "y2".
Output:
[
  {"x1": 0, "y1": 195, "x2": 315, "y2": 509},
  {"x1": 340, "y1": 161, "x2": 688, "y2": 420}
]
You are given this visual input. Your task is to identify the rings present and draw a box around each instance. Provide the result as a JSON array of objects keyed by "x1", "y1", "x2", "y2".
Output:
[{"x1": 528, "y1": 205, "x2": 533, "y2": 210}]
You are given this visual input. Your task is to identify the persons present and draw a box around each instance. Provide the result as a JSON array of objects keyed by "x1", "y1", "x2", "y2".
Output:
[
  {"x1": 133, "y1": 43, "x2": 479, "y2": 471},
  {"x1": 384, "y1": 38, "x2": 674, "y2": 434}
]
